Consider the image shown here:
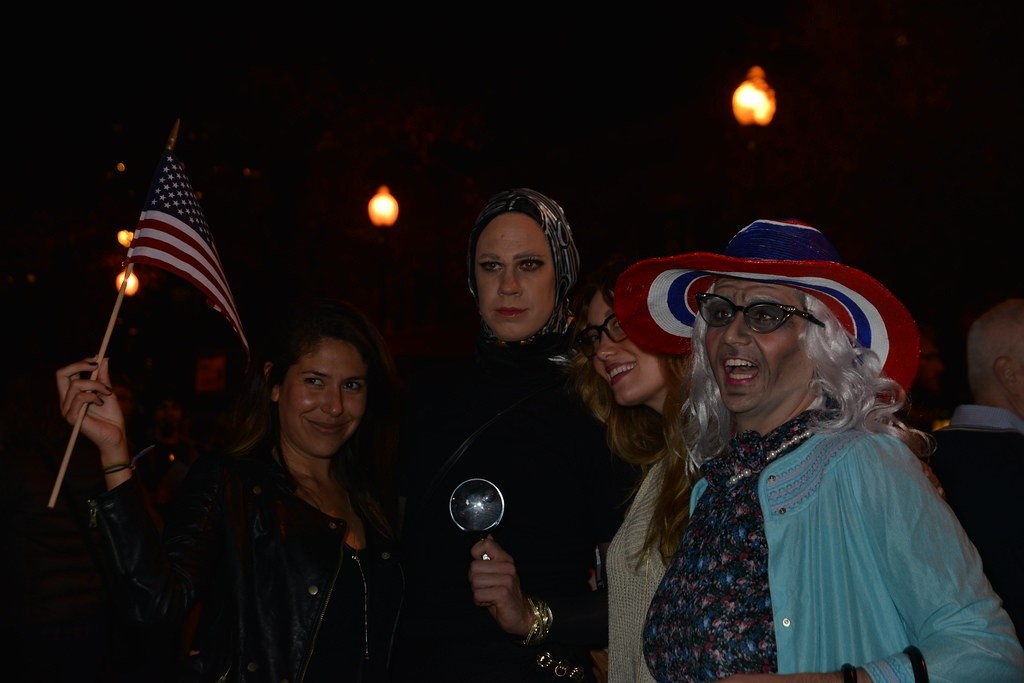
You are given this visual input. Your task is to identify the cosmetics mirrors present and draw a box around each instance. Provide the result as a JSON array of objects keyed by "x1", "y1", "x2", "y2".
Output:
[{"x1": 449, "y1": 477, "x2": 505, "y2": 563}]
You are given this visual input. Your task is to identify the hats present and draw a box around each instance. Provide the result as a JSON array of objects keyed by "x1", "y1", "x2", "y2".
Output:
[{"x1": 613, "y1": 219, "x2": 922, "y2": 404}]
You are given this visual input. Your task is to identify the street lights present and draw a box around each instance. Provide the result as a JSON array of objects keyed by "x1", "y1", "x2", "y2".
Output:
[
  {"x1": 364, "y1": 183, "x2": 399, "y2": 335},
  {"x1": 726, "y1": 65, "x2": 777, "y2": 226}
]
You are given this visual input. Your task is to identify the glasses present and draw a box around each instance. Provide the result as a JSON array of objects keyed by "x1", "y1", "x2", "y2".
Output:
[
  {"x1": 695, "y1": 290, "x2": 825, "y2": 334},
  {"x1": 576, "y1": 313, "x2": 629, "y2": 357}
]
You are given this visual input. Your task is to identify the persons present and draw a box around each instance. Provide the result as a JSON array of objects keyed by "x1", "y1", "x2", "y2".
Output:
[
  {"x1": 55, "y1": 295, "x2": 405, "y2": 683},
  {"x1": 394, "y1": 186, "x2": 646, "y2": 683},
  {"x1": 569, "y1": 256, "x2": 702, "y2": 682},
  {"x1": 613, "y1": 219, "x2": 1024, "y2": 683},
  {"x1": 923, "y1": 295, "x2": 1023, "y2": 650}
]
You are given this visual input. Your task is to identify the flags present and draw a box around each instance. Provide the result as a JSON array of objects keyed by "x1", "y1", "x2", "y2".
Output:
[{"x1": 123, "y1": 150, "x2": 250, "y2": 372}]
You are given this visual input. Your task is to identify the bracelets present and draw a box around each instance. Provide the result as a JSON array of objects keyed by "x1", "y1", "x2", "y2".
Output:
[
  {"x1": 102, "y1": 461, "x2": 136, "y2": 474},
  {"x1": 512, "y1": 595, "x2": 551, "y2": 647},
  {"x1": 840, "y1": 664, "x2": 857, "y2": 683}
]
